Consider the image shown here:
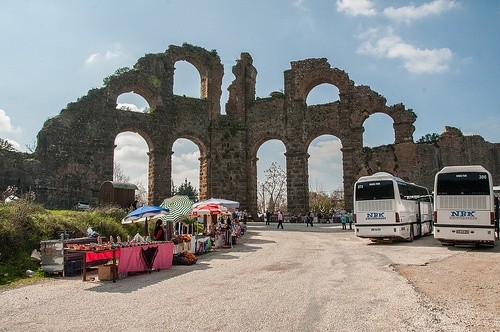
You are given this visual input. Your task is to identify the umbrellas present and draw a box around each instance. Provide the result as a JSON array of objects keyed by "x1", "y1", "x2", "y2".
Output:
[{"x1": 121, "y1": 195, "x2": 240, "y2": 236}]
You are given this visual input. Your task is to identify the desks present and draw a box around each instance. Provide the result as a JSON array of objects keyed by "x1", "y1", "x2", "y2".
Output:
[{"x1": 64, "y1": 241, "x2": 174, "y2": 283}]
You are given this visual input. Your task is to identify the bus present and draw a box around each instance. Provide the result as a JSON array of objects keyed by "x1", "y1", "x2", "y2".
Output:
[
  {"x1": 433, "y1": 164, "x2": 495, "y2": 247},
  {"x1": 352, "y1": 172, "x2": 436, "y2": 242}
]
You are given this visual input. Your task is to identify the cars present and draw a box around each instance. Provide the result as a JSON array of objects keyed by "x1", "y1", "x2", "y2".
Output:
[{"x1": 72, "y1": 201, "x2": 91, "y2": 210}]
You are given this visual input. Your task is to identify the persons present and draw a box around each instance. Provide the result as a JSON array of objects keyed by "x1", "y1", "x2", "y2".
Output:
[
  {"x1": 232, "y1": 208, "x2": 353, "y2": 230},
  {"x1": 153, "y1": 220, "x2": 165, "y2": 240}
]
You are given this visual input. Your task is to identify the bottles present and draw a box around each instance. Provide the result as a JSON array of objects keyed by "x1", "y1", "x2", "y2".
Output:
[
  {"x1": 117, "y1": 235, "x2": 120, "y2": 242},
  {"x1": 127, "y1": 235, "x2": 130, "y2": 243},
  {"x1": 110, "y1": 236, "x2": 113, "y2": 244},
  {"x1": 97, "y1": 236, "x2": 102, "y2": 245}
]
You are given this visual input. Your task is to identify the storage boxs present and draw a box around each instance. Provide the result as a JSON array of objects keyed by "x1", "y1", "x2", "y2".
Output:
[{"x1": 97, "y1": 265, "x2": 118, "y2": 280}]
